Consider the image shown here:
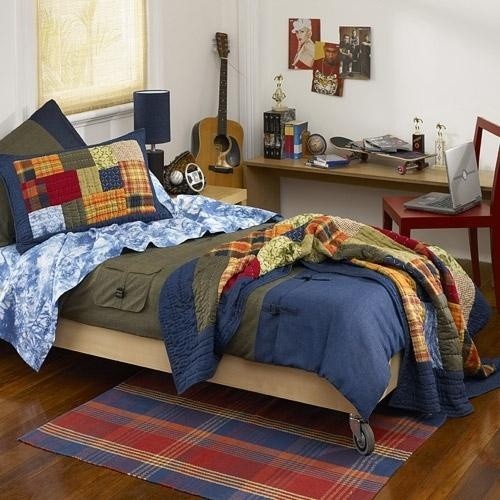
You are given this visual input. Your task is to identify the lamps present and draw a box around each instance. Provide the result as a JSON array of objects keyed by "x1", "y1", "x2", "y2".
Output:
[{"x1": 134, "y1": 91, "x2": 170, "y2": 183}]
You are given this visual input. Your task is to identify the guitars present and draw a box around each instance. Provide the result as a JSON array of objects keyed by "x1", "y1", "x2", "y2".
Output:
[{"x1": 192, "y1": 33, "x2": 243, "y2": 188}]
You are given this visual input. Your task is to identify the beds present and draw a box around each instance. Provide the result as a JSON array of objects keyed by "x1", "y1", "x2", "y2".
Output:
[{"x1": 0, "y1": 195, "x2": 459, "y2": 455}]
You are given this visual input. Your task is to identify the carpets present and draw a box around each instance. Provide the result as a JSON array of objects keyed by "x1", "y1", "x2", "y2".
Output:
[{"x1": 19, "y1": 358, "x2": 499, "y2": 499}]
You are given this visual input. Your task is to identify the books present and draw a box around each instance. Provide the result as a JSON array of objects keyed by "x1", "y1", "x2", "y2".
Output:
[
  {"x1": 362, "y1": 138, "x2": 397, "y2": 152},
  {"x1": 310, "y1": 158, "x2": 346, "y2": 168},
  {"x1": 311, "y1": 154, "x2": 349, "y2": 164},
  {"x1": 364, "y1": 134, "x2": 411, "y2": 151},
  {"x1": 263, "y1": 108, "x2": 308, "y2": 159}
]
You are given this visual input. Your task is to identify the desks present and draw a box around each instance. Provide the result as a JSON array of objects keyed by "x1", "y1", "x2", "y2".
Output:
[{"x1": 244, "y1": 150, "x2": 494, "y2": 213}]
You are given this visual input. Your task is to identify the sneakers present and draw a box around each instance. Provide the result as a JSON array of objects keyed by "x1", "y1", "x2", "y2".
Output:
[
  {"x1": 347, "y1": 72, "x2": 354, "y2": 77},
  {"x1": 339, "y1": 73, "x2": 345, "y2": 77}
]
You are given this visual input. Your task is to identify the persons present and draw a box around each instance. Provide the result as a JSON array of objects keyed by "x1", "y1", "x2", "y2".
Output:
[
  {"x1": 339, "y1": 34, "x2": 353, "y2": 77},
  {"x1": 291, "y1": 19, "x2": 315, "y2": 70},
  {"x1": 350, "y1": 30, "x2": 360, "y2": 60},
  {"x1": 312, "y1": 42, "x2": 344, "y2": 97},
  {"x1": 360, "y1": 34, "x2": 371, "y2": 58}
]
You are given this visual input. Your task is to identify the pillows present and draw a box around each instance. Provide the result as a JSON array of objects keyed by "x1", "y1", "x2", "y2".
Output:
[
  {"x1": 0, "y1": 102, "x2": 87, "y2": 246},
  {"x1": 7, "y1": 139, "x2": 174, "y2": 250}
]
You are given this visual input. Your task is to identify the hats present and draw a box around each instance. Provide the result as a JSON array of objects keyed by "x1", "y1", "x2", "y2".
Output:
[
  {"x1": 323, "y1": 42, "x2": 339, "y2": 49},
  {"x1": 292, "y1": 19, "x2": 311, "y2": 33}
]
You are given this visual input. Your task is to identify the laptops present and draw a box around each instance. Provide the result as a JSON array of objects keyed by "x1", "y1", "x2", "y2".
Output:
[{"x1": 404, "y1": 142, "x2": 483, "y2": 216}]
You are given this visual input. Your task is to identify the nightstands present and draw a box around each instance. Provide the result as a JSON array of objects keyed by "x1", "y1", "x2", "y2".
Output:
[{"x1": 197, "y1": 186, "x2": 248, "y2": 209}]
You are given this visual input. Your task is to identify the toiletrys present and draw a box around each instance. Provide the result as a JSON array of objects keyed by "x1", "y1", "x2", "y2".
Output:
[
  {"x1": 434, "y1": 123, "x2": 446, "y2": 167},
  {"x1": 412, "y1": 117, "x2": 425, "y2": 164}
]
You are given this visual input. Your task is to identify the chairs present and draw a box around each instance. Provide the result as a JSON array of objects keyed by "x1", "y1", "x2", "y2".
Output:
[{"x1": 383, "y1": 117, "x2": 499, "y2": 308}]
